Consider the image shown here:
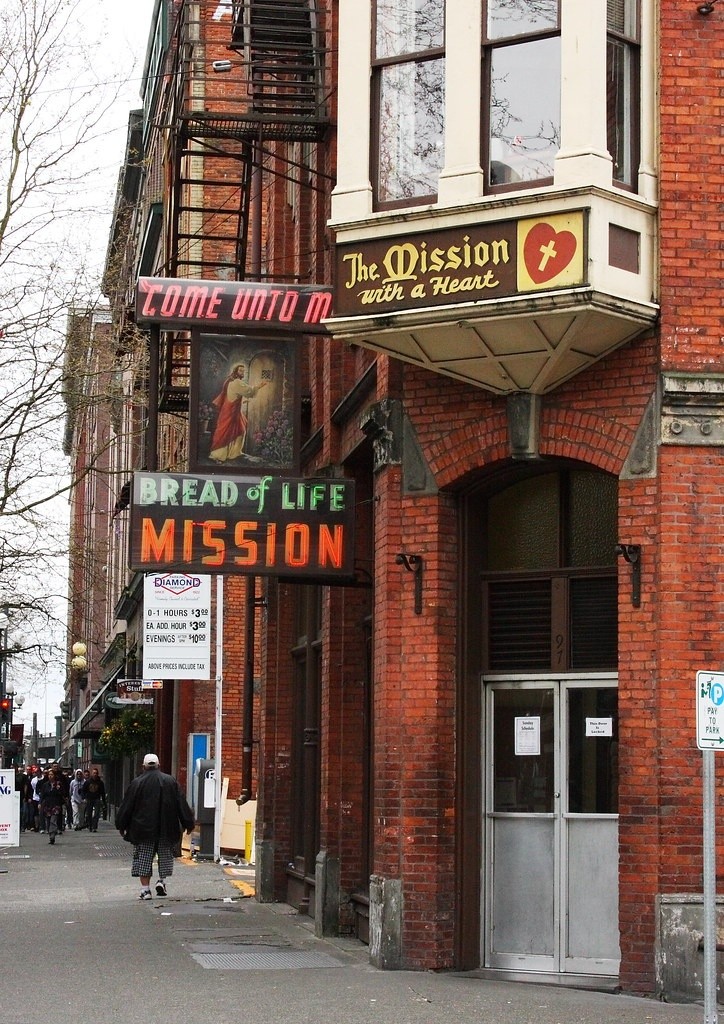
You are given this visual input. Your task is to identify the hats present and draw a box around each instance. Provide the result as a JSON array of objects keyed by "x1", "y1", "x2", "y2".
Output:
[{"x1": 142, "y1": 754, "x2": 159, "y2": 766}]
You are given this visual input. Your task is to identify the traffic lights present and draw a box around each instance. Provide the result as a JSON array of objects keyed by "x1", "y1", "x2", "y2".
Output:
[{"x1": 1, "y1": 698, "x2": 12, "y2": 723}]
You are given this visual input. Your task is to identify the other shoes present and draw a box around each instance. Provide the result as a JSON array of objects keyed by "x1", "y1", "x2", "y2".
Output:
[
  {"x1": 59, "y1": 831, "x2": 62, "y2": 834},
  {"x1": 30, "y1": 827, "x2": 35, "y2": 831},
  {"x1": 93, "y1": 829, "x2": 96, "y2": 832},
  {"x1": 155, "y1": 880, "x2": 167, "y2": 896},
  {"x1": 39, "y1": 830, "x2": 44, "y2": 834},
  {"x1": 139, "y1": 890, "x2": 152, "y2": 900},
  {"x1": 49, "y1": 838, "x2": 55, "y2": 845}
]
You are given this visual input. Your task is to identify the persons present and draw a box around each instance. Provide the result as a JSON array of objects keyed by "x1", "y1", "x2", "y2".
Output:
[
  {"x1": 116, "y1": 753, "x2": 194, "y2": 899},
  {"x1": 11, "y1": 762, "x2": 105, "y2": 845}
]
